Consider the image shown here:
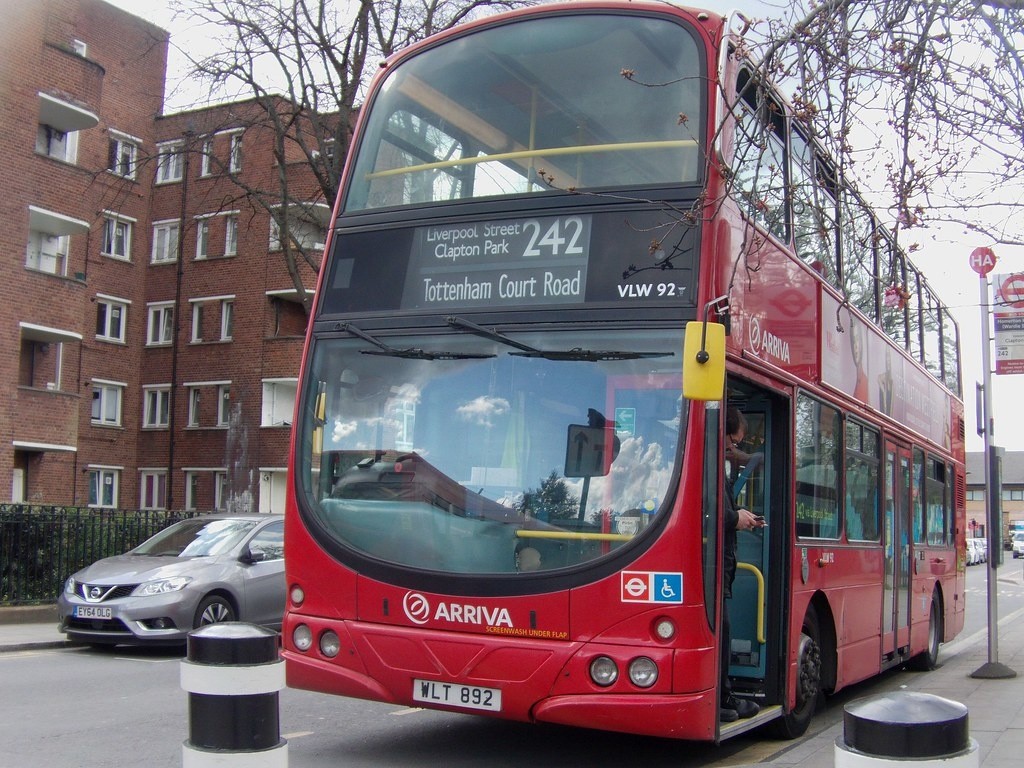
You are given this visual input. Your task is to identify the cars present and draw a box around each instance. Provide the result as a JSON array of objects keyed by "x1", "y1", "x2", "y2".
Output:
[
  {"x1": 55, "y1": 512, "x2": 286, "y2": 651},
  {"x1": 966, "y1": 537, "x2": 987, "y2": 566}
]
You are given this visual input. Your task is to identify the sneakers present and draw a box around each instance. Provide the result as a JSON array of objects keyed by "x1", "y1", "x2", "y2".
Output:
[
  {"x1": 719, "y1": 708, "x2": 739, "y2": 722},
  {"x1": 720, "y1": 693, "x2": 760, "y2": 718}
]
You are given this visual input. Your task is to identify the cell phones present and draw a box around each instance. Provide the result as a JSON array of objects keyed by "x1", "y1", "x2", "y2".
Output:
[{"x1": 755, "y1": 516, "x2": 765, "y2": 522}]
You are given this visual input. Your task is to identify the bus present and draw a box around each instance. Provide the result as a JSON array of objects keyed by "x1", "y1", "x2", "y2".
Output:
[
  {"x1": 319, "y1": 447, "x2": 580, "y2": 575},
  {"x1": 279, "y1": 0, "x2": 967, "y2": 747},
  {"x1": 795, "y1": 461, "x2": 951, "y2": 588}
]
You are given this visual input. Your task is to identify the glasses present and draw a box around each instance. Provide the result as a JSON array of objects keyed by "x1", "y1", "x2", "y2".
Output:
[{"x1": 729, "y1": 433, "x2": 739, "y2": 448}]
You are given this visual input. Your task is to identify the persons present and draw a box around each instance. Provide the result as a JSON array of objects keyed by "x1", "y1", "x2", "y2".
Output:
[{"x1": 719, "y1": 407, "x2": 765, "y2": 723}]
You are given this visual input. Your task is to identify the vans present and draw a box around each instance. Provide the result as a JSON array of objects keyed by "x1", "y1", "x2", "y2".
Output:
[{"x1": 1012, "y1": 532, "x2": 1024, "y2": 558}]
